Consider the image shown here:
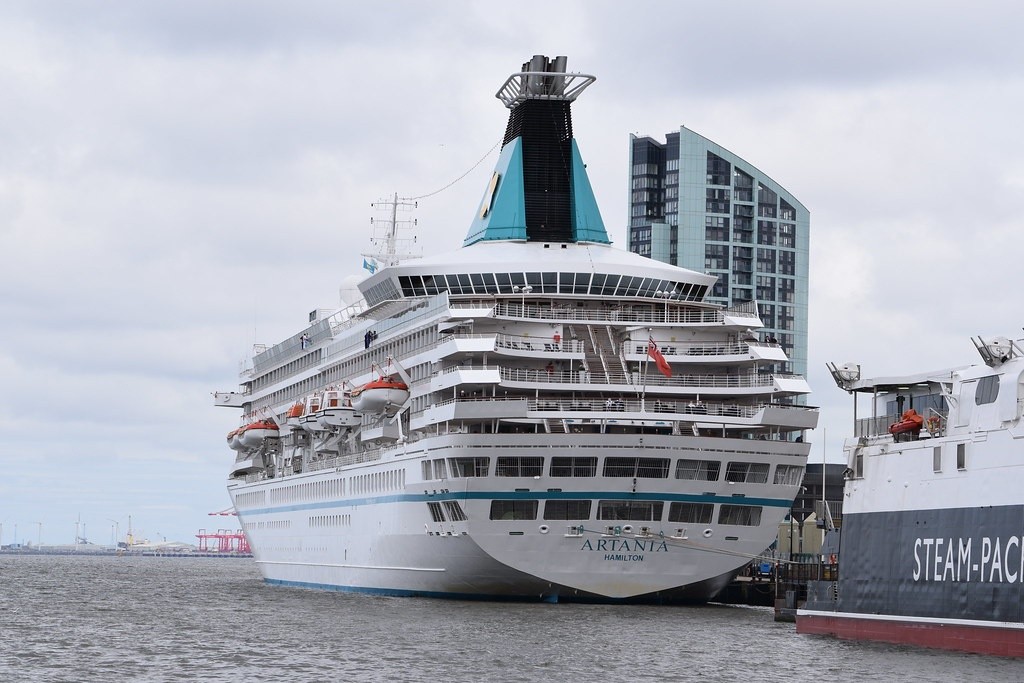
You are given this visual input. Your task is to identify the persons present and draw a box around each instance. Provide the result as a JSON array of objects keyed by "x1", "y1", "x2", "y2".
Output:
[
  {"x1": 795, "y1": 434, "x2": 803, "y2": 443},
  {"x1": 535, "y1": 399, "x2": 743, "y2": 417},
  {"x1": 760, "y1": 434, "x2": 766, "y2": 441},
  {"x1": 300, "y1": 333, "x2": 308, "y2": 351},
  {"x1": 553, "y1": 330, "x2": 561, "y2": 347},
  {"x1": 364, "y1": 330, "x2": 379, "y2": 349},
  {"x1": 764, "y1": 335, "x2": 777, "y2": 348}
]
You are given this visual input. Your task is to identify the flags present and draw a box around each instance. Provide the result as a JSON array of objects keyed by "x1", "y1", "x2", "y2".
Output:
[
  {"x1": 648, "y1": 335, "x2": 672, "y2": 378},
  {"x1": 363, "y1": 258, "x2": 379, "y2": 274}
]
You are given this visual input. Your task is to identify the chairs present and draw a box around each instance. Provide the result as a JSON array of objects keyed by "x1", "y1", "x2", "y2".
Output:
[
  {"x1": 543, "y1": 343, "x2": 560, "y2": 352},
  {"x1": 636, "y1": 346, "x2": 678, "y2": 356},
  {"x1": 687, "y1": 348, "x2": 725, "y2": 355},
  {"x1": 497, "y1": 341, "x2": 533, "y2": 350}
]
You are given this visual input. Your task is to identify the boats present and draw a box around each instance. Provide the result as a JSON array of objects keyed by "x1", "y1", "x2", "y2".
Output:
[{"x1": 796, "y1": 327, "x2": 1024, "y2": 659}]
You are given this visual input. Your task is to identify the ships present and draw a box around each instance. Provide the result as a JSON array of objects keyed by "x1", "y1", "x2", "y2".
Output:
[{"x1": 208, "y1": 54, "x2": 821, "y2": 601}]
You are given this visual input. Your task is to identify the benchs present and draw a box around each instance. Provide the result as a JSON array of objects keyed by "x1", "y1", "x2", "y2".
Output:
[
  {"x1": 603, "y1": 402, "x2": 625, "y2": 411},
  {"x1": 654, "y1": 402, "x2": 677, "y2": 413},
  {"x1": 538, "y1": 402, "x2": 559, "y2": 411},
  {"x1": 686, "y1": 405, "x2": 708, "y2": 414},
  {"x1": 570, "y1": 403, "x2": 591, "y2": 410},
  {"x1": 718, "y1": 407, "x2": 742, "y2": 417}
]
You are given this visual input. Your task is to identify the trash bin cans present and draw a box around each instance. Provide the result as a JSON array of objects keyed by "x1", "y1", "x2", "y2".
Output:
[{"x1": 761, "y1": 564, "x2": 770, "y2": 573}]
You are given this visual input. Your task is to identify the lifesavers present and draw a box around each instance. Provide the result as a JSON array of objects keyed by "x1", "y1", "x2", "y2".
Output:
[{"x1": 926, "y1": 416, "x2": 942, "y2": 433}]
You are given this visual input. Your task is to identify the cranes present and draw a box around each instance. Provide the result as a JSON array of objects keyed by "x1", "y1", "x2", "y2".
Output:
[{"x1": 125, "y1": 515, "x2": 133, "y2": 546}]
